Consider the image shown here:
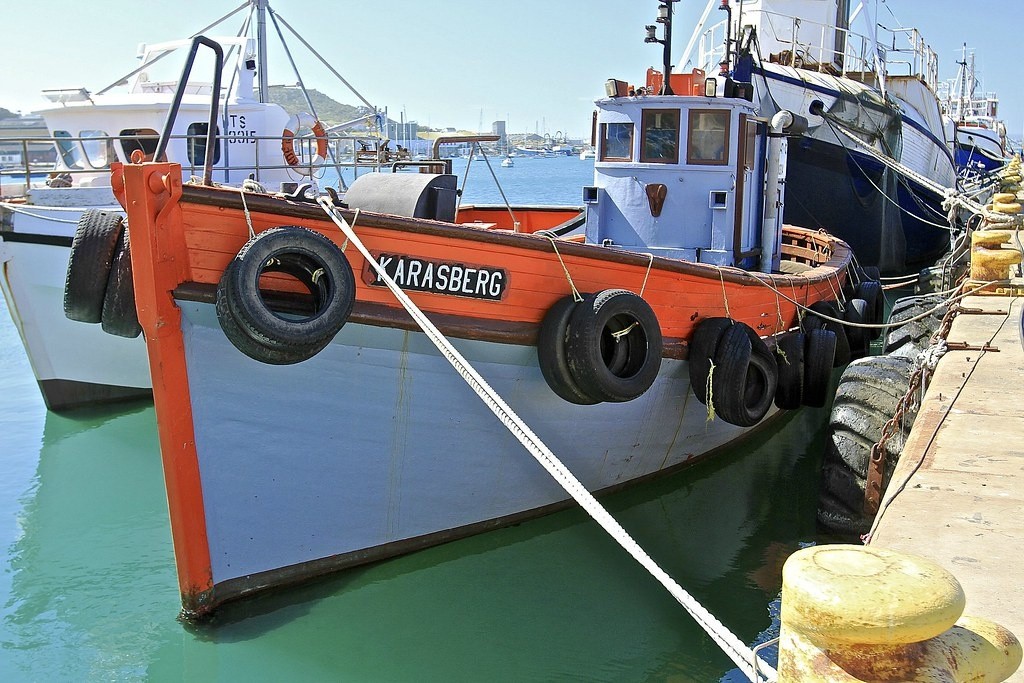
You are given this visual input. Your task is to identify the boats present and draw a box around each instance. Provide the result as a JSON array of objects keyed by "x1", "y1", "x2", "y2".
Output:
[
  {"x1": 502, "y1": 157, "x2": 514, "y2": 167},
  {"x1": 935, "y1": 43, "x2": 1007, "y2": 225},
  {"x1": 109, "y1": 0, "x2": 856, "y2": 620},
  {"x1": 674, "y1": 0, "x2": 959, "y2": 293},
  {"x1": 0, "y1": 0, "x2": 501, "y2": 412}
]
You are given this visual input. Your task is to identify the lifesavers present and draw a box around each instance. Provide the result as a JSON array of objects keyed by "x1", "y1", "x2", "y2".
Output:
[
  {"x1": 687, "y1": 315, "x2": 781, "y2": 429},
  {"x1": 535, "y1": 286, "x2": 667, "y2": 406},
  {"x1": 772, "y1": 328, "x2": 838, "y2": 411},
  {"x1": 805, "y1": 264, "x2": 885, "y2": 368},
  {"x1": 58, "y1": 206, "x2": 143, "y2": 342},
  {"x1": 282, "y1": 111, "x2": 329, "y2": 177},
  {"x1": 212, "y1": 222, "x2": 358, "y2": 367}
]
